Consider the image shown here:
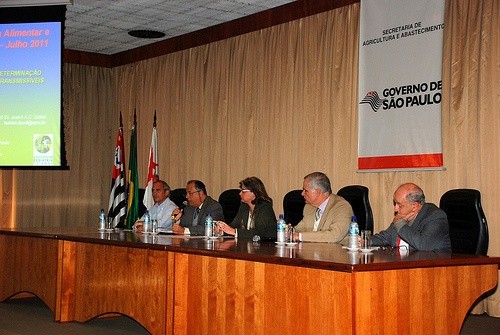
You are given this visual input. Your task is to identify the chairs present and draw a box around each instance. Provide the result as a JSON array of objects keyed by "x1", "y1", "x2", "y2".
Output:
[{"x1": 169, "y1": 185, "x2": 490, "y2": 264}]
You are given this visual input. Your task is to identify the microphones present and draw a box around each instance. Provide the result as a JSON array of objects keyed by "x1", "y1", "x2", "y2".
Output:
[{"x1": 171, "y1": 201, "x2": 188, "y2": 220}]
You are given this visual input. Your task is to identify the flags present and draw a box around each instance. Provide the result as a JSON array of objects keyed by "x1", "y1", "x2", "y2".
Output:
[
  {"x1": 142, "y1": 126, "x2": 158, "y2": 212},
  {"x1": 107, "y1": 127, "x2": 127, "y2": 229},
  {"x1": 123, "y1": 123, "x2": 138, "y2": 231}
]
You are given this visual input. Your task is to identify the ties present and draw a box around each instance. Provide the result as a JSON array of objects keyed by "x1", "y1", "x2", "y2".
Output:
[
  {"x1": 315, "y1": 208, "x2": 321, "y2": 221},
  {"x1": 192, "y1": 207, "x2": 200, "y2": 226}
]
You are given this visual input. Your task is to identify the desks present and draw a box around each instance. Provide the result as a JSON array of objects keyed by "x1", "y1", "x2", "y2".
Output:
[{"x1": 0, "y1": 227, "x2": 500, "y2": 335}]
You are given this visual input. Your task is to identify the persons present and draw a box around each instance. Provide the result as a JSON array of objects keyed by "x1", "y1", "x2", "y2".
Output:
[
  {"x1": 132, "y1": 180, "x2": 180, "y2": 234},
  {"x1": 284, "y1": 172, "x2": 358, "y2": 245},
  {"x1": 171, "y1": 180, "x2": 226, "y2": 236},
  {"x1": 358, "y1": 183, "x2": 452, "y2": 253},
  {"x1": 214, "y1": 176, "x2": 277, "y2": 241}
]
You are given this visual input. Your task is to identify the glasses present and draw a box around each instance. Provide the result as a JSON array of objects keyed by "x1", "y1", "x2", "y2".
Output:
[
  {"x1": 241, "y1": 189, "x2": 251, "y2": 193},
  {"x1": 188, "y1": 190, "x2": 199, "y2": 196}
]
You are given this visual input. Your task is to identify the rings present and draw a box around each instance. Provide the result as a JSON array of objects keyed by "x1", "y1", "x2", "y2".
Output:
[{"x1": 220, "y1": 226, "x2": 222, "y2": 228}]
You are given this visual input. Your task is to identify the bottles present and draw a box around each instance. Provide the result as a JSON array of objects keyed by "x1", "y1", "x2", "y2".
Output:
[
  {"x1": 99, "y1": 209, "x2": 105, "y2": 230},
  {"x1": 205, "y1": 212, "x2": 213, "y2": 236},
  {"x1": 277, "y1": 214, "x2": 285, "y2": 242},
  {"x1": 143, "y1": 210, "x2": 150, "y2": 232},
  {"x1": 348, "y1": 216, "x2": 359, "y2": 248}
]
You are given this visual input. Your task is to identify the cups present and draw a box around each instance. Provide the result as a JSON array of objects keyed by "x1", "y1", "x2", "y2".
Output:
[
  {"x1": 106, "y1": 217, "x2": 113, "y2": 229},
  {"x1": 213, "y1": 222, "x2": 220, "y2": 237},
  {"x1": 286, "y1": 228, "x2": 296, "y2": 243},
  {"x1": 150, "y1": 219, "x2": 158, "y2": 233},
  {"x1": 361, "y1": 230, "x2": 371, "y2": 249}
]
